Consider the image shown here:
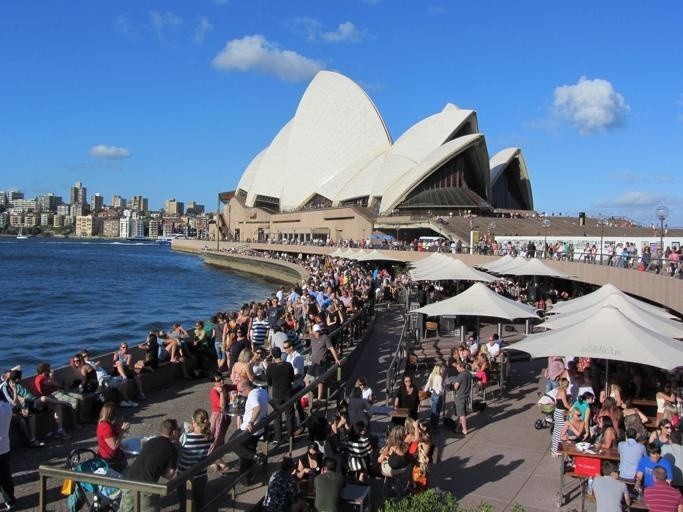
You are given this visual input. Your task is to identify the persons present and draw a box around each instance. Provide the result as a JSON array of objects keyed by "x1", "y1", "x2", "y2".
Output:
[
  {"x1": 1, "y1": 319, "x2": 208, "y2": 512},
  {"x1": 197, "y1": 205, "x2": 682, "y2": 511}
]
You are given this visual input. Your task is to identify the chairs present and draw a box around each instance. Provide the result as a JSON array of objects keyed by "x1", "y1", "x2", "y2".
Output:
[
  {"x1": 417, "y1": 351, "x2": 510, "y2": 434},
  {"x1": 424, "y1": 321, "x2": 439, "y2": 338}
]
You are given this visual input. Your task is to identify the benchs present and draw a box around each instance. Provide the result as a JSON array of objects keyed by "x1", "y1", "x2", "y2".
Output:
[{"x1": 9, "y1": 329, "x2": 196, "y2": 449}]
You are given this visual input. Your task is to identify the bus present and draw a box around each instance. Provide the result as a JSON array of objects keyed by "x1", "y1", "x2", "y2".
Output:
[{"x1": 419, "y1": 236, "x2": 445, "y2": 250}]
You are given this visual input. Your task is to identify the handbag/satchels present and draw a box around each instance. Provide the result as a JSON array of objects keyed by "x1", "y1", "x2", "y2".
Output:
[{"x1": 60, "y1": 458, "x2": 75, "y2": 496}]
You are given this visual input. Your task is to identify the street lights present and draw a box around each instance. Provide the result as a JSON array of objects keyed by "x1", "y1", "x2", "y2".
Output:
[
  {"x1": 542, "y1": 220, "x2": 551, "y2": 260},
  {"x1": 656, "y1": 205, "x2": 668, "y2": 274},
  {"x1": 598, "y1": 210, "x2": 607, "y2": 264}
]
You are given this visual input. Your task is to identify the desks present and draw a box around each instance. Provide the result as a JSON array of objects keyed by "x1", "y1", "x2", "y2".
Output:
[
  {"x1": 554, "y1": 351, "x2": 659, "y2": 511},
  {"x1": 297, "y1": 405, "x2": 411, "y2": 511}
]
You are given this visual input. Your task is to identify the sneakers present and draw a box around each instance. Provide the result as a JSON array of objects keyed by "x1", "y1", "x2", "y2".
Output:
[
  {"x1": 128, "y1": 400, "x2": 138, "y2": 407},
  {"x1": 137, "y1": 393, "x2": 146, "y2": 400},
  {"x1": 54, "y1": 430, "x2": 72, "y2": 440},
  {"x1": 118, "y1": 400, "x2": 131, "y2": 407},
  {"x1": 0, "y1": 501, "x2": 16, "y2": 512},
  {"x1": 28, "y1": 438, "x2": 45, "y2": 446}
]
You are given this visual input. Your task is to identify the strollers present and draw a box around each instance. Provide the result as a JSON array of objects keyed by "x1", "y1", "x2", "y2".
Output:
[
  {"x1": 535, "y1": 391, "x2": 574, "y2": 434},
  {"x1": 64, "y1": 447, "x2": 128, "y2": 512}
]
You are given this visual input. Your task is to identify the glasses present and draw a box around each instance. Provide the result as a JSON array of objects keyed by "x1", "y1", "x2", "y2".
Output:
[{"x1": 217, "y1": 378, "x2": 223, "y2": 382}]
